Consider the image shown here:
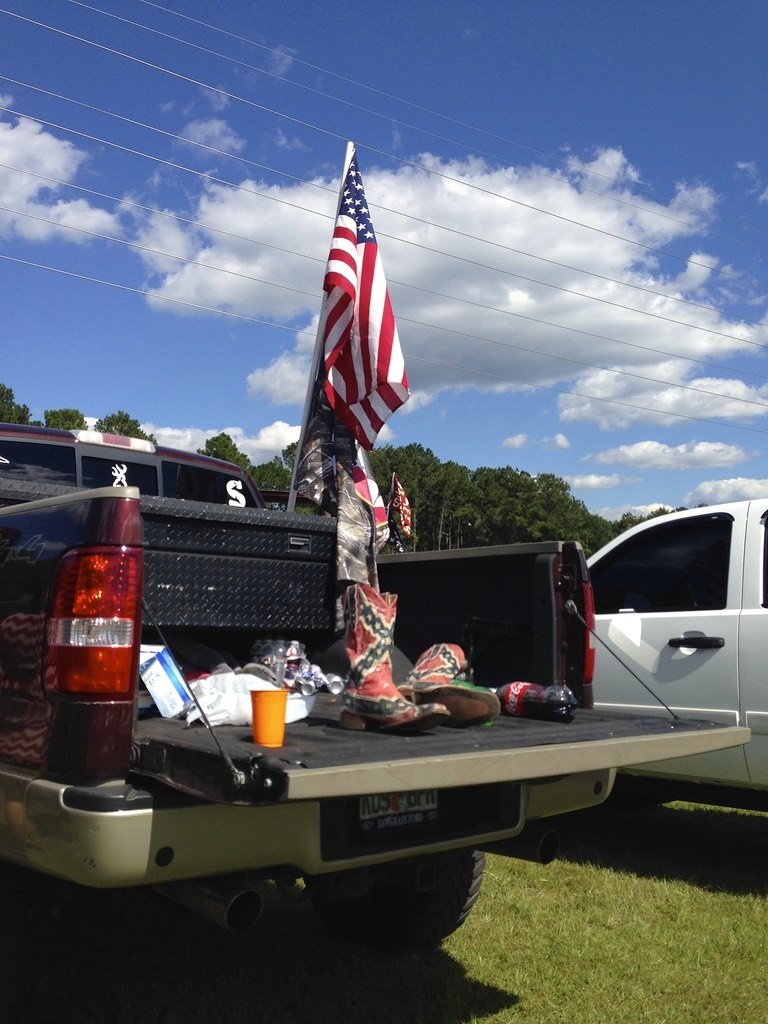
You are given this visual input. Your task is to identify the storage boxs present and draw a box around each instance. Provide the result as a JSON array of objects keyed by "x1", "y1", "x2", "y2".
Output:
[{"x1": 141, "y1": 642, "x2": 194, "y2": 718}]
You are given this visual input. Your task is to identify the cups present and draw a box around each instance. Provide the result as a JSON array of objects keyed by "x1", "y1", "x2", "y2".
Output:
[{"x1": 250, "y1": 690, "x2": 289, "y2": 748}]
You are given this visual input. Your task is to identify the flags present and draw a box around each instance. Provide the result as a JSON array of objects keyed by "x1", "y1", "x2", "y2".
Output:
[{"x1": 322, "y1": 148, "x2": 411, "y2": 454}]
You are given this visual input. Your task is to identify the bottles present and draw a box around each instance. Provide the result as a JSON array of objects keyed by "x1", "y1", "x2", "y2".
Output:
[
  {"x1": 495, "y1": 682, "x2": 579, "y2": 724},
  {"x1": 249, "y1": 639, "x2": 300, "y2": 688}
]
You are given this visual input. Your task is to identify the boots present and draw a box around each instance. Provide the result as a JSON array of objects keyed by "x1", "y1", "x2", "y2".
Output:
[
  {"x1": 339, "y1": 584, "x2": 450, "y2": 731},
  {"x1": 396, "y1": 642, "x2": 501, "y2": 727}
]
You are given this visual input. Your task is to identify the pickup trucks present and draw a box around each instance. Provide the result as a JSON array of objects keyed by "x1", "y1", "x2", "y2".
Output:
[
  {"x1": 1, "y1": 423, "x2": 753, "y2": 952},
  {"x1": 582, "y1": 500, "x2": 767, "y2": 793}
]
